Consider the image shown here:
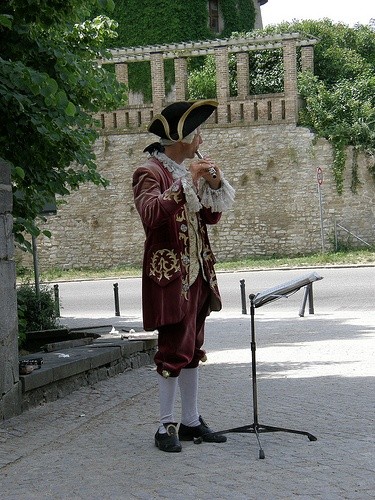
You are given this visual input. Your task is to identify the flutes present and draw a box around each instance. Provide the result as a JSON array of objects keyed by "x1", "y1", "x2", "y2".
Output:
[{"x1": 196, "y1": 150, "x2": 217, "y2": 180}]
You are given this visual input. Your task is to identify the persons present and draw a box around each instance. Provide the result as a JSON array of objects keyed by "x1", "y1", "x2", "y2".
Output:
[{"x1": 132, "y1": 100, "x2": 235, "y2": 452}]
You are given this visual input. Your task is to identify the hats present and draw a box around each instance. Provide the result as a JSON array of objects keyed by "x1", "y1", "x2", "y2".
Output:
[{"x1": 147, "y1": 100, "x2": 219, "y2": 142}]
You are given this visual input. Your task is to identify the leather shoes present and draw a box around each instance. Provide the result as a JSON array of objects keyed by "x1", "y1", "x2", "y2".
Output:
[
  {"x1": 178, "y1": 415, "x2": 228, "y2": 443},
  {"x1": 155, "y1": 422, "x2": 181, "y2": 452}
]
